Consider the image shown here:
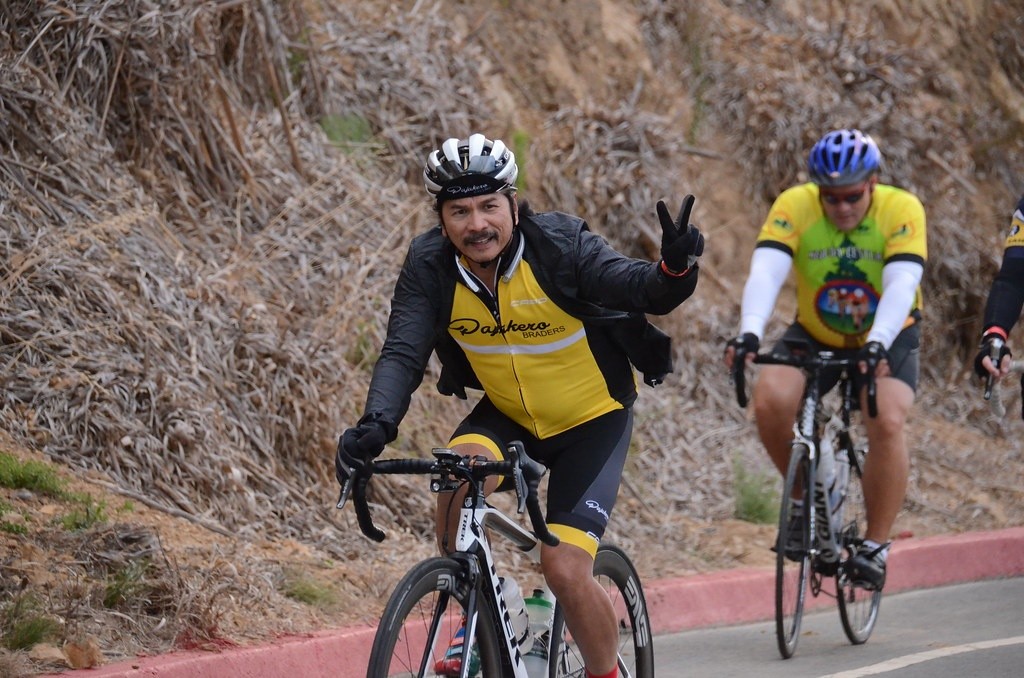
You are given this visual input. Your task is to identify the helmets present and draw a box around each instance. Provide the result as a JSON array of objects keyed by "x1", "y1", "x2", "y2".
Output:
[
  {"x1": 422, "y1": 133, "x2": 518, "y2": 200},
  {"x1": 807, "y1": 129, "x2": 881, "y2": 187}
]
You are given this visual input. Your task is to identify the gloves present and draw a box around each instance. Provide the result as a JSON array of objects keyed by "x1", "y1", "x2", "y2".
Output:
[
  {"x1": 334, "y1": 419, "x2": 387, "y2": 487},
  {"x1": 656, "y1": 194, "x2": 704, "y2": 275}
]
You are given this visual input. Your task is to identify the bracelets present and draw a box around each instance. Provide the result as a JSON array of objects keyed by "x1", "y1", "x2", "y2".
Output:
[
  {"x1": 980, "y1": 325, "x2": 1007, "y2": 344},
  {"x1": 659, "y1": 261, "x2": 689, "y2": 276}
]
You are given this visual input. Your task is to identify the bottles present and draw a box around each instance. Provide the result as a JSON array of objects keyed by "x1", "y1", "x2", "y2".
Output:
[
  {"x1": 829, "y1": 448, "x2": 850, "y2": 504},
  {"x1": 520, "y1": 588, "x2": 555, "y2": 678},
  {"x1": 818, "y1": 436, "x2": 837, "y2": 483},
  {"x1": 498, "y1": 575, "x2": 535, "y2": 656}
]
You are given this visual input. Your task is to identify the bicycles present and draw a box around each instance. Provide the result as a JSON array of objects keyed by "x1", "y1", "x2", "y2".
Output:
[
  {"x1": 729, "y1": 339, "x2": 887, "y2": 659},
  {"x1": 977, "y1": 338, "x2": 1024, "y2": 423},
  {"x1": 336, "y1": 440, "x2": 655, "y2": 678}
]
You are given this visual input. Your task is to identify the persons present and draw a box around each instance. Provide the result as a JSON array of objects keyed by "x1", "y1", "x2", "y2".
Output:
[
  {"x1": 334, "y1": 129, "x2": 707, "y2": 678},
  {"x1": 722, "y1": 129, "x2": 929, "y2": 592},
  {"x1": 973, "y1": 187, "x2": 1024, "y2": 421}
]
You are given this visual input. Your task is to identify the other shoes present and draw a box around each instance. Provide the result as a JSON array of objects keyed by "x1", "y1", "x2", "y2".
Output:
[
  {"x1": 849, "y1": 542, "x2": 892, "y2": 592},
  {"x1": 433, "y1": 610, "x2": 481, "y2": 678},
  {"x1": 768, "y1": 505, "x2": 805, "y2": 562}
]
